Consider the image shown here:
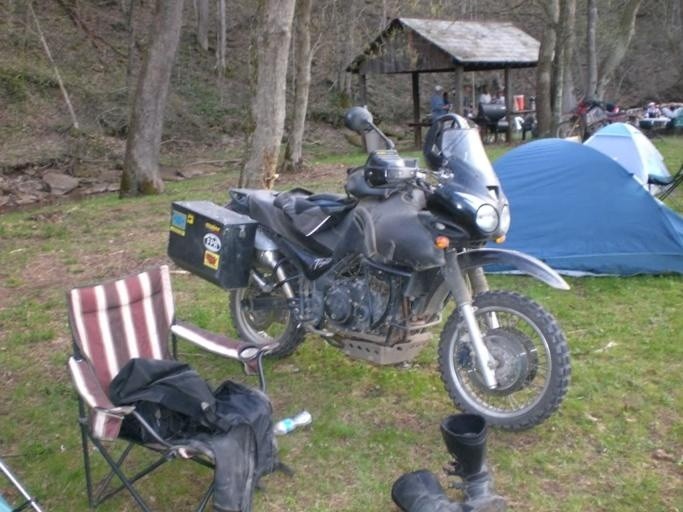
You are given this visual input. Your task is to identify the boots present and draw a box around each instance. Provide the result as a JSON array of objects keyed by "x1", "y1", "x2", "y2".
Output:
[
  {"x1": 438, "y1": 413, "x2": 495, "y2": 505},
  {"x1": 393, "y1": 468, "x2": 466, "y2": 512}
]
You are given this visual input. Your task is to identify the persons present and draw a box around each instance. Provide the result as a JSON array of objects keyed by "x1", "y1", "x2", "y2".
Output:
[{"x1": 432, "y1": 83, "x2": 683, "y2": 144}]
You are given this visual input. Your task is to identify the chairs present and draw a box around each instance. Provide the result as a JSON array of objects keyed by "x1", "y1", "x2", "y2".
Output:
[{"x1": 64, "y1": 264, "x2": 292, "y2": 509}]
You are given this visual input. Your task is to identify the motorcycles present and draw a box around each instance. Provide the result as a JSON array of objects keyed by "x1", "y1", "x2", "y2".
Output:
[
  {"x1": 557, "y1": 92, "x2": 627, "y2": 144},
  {"x1": 168, "y1": 102, "x2": 573, "y2": 433}
]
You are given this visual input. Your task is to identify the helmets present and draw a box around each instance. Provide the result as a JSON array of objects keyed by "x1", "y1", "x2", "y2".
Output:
[{"x1": 423, "y1": 111, "x2": 484, "y2": 185}]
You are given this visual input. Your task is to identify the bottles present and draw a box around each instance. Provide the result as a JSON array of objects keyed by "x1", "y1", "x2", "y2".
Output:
[{"x1": 272, "y1": 411, "x2": 312, "y2": 436}]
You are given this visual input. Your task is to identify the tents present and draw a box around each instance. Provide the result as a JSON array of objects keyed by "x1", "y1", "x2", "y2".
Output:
[
  {"x1": 479, "y1": 137, "x2": 683, "y2": 279},
  {"x1": 581, "y1": 121, "x2": 674, "y2": 196}
]
